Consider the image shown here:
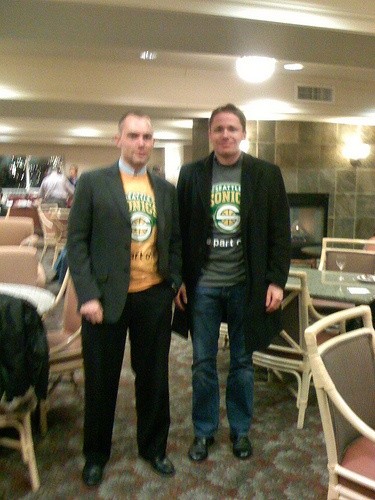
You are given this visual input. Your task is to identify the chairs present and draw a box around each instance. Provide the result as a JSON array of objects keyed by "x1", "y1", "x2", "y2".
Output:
[
  {"x1": 40, "y1": 264, "x2": 85, "y2": 434},
  {"x1": 318, "y1": 237, "x2": 375, "y2": 275},
  {"x1": 0, "y1": 293, "x2": 49, "y2": 493},
  {"x1": 304, "y1": 304, "x2": 375, "y2": 500},
  {"x1": 252, "y1": 269, "x2": 346, "y2": 430},
  {"x1": 37, "y1": 203, "x2": 71, "y2": 270}
]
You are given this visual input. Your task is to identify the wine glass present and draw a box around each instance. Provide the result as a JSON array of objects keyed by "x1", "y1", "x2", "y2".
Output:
[{"x1": 336, "y1": 253, "x2": 348, "y2": 281}]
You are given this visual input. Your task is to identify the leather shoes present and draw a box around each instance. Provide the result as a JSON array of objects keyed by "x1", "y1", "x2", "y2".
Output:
[
  {"x1": 230, "y1": 431, "x2": 252, "y2": 461},
  {"x1": 138, "y1": 452, "x2": 175, "y2": 478},
  {"x1": 187, "y1": 436, "x2": 214, "y2": 461},
  {"x1": 80, "y1": 458, "x2": 108, "y2": 487}
]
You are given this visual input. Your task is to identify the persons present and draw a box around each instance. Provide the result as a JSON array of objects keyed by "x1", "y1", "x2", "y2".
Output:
[
  {"x1": 172, "y1": 105, "x2": 291, "y2": 462},
  {"x1": 66, "y1": 109, "x2": 182, "y2": 488},
  {"x1": 39, "y1": 156, "x2": 79, "y2": 206}
]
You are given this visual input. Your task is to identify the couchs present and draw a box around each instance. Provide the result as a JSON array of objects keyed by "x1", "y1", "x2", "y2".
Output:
[
  {"x1": 0, "y1": 217, "x2": 38, "y2": 246},
  {"x1": 0, "y1": 245, "x2": 46, "y2": 288}
]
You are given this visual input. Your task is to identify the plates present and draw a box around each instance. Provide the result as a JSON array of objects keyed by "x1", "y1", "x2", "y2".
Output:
[{"x1": 357, "y1": 273, "x2": 375, "y2": 283}]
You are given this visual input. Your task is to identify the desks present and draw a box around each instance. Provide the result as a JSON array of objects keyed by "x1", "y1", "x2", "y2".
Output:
[
  {"x1": 0, "y1": 283, "x2": 56, "y2": 316},
  {"x1": 285, "y1": 267, "x2": 375, "y2": 303}
]
